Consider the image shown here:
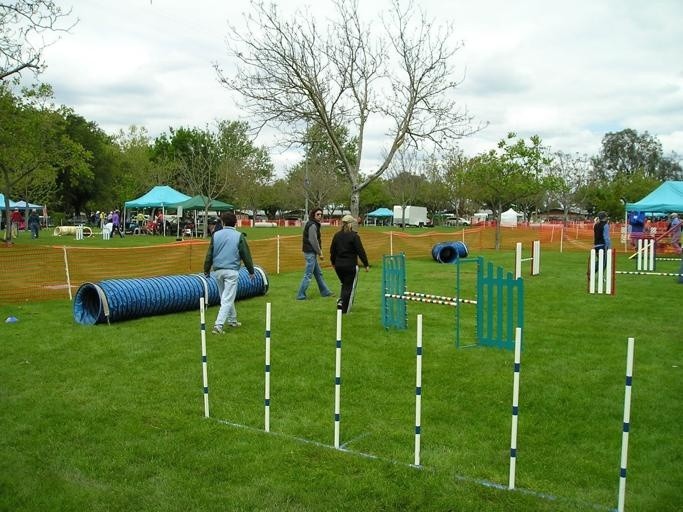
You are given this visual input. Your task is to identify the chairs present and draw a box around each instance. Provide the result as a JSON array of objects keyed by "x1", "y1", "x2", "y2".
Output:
[{"x1": 116, "y1": 217, "x2": 222, "y2": 241}]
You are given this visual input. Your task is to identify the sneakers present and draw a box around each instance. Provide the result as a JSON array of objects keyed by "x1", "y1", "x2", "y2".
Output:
[{"x1": 212, "y1": 322, "x2": 242, "y2": 334}]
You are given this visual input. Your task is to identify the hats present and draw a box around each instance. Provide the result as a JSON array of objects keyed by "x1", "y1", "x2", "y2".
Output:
[{"x1": 342, "y1": 215, "x2": 356, "y2": 223}]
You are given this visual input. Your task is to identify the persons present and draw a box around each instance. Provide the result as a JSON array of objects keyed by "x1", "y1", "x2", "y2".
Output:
[
  {"x1": 670, "y1": 212, "x2": 683, "y2": 256},
  {"x1": 28, "y1": 210, "x2": 42, "y2": 239},
  {"x1": 593, "y1": 212, "x2": 611, "y2": 282},
  {"x1": 627, "y1": 225, "x2": 632, "y2": 241},
  {"x1": 94, "y1": 209, "x2": 124, "y2": 239},
  {"x1": 646, "y1": 219, "x2": 651, "y2": 229},
  {"x1": 204, "y1": 211, "x2": 255, "y2": 335},
  {"x1": 10, "y1": 208, "x2": 24, "y2": 238},
  {"x1": 154, "y1": 209, "x2": 163, "y2": 234},
  {"x1": 136, "y1": 211, "x2": 145, "y2": 226},
  {"x1": 329, "y1": 215, "x2": 370, "y2": 314},
  {"x1": 296, "y1": 206, "x2": 333, "y2": 300}
]
